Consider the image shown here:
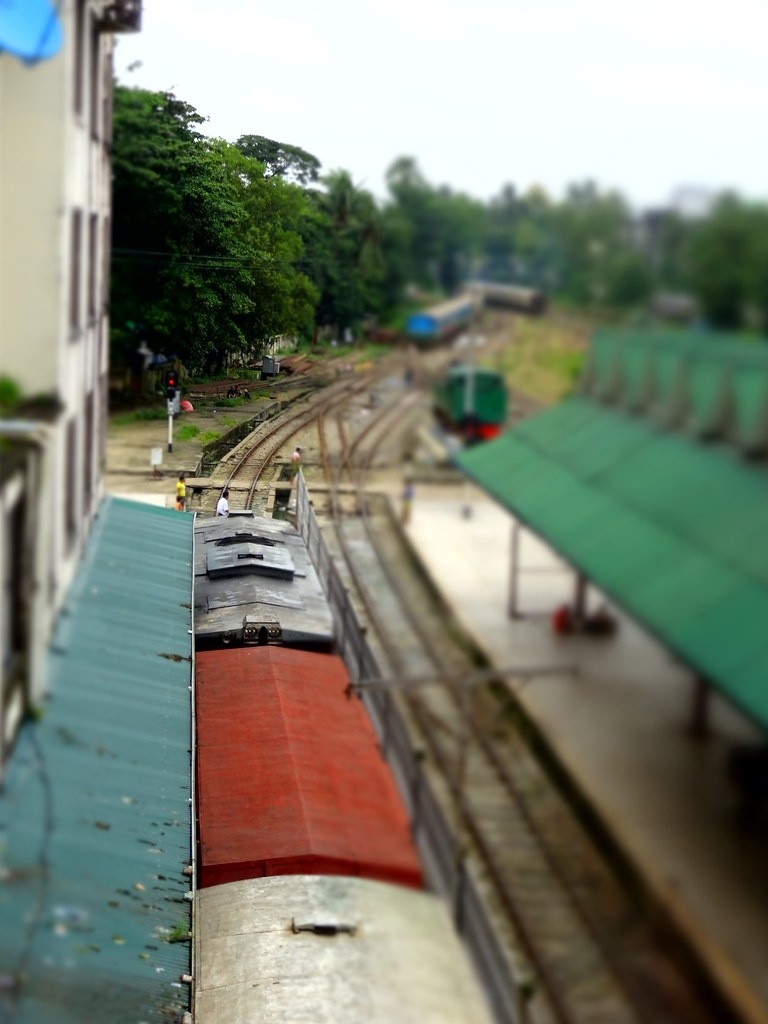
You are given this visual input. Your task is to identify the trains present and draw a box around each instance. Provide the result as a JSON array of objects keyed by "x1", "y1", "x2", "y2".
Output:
[
  {"x1": 402, "y1": 286, "x2": 487, "y2": 349},
  {"x1": 431, "y1": 360, "x2": 507, "y2": 444},
  {"x1": 464, "y1": 281, "x2": 547, "y2": 314}
]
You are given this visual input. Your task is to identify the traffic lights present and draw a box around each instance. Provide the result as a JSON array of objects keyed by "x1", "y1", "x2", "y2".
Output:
[{"x1": 165, "y1": 371, "x2": 177, "y2": 399}]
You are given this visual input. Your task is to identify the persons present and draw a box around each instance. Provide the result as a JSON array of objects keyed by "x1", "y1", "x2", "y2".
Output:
[
  {"x1": 175, "y1": 475, "x2": 186, "y2": 512},
  {"x1": 226, "y1": 386, "x2": 251, "y2": 399},
  {"x1": 292, "y1": 446, "x2": 303, "y2": 478},
  {"x1": 233, "y1": 358, "x2": 243, "y2": 369},
  {"x1": 215, "y1": 491, "x2": 230, "y2": 518}
]
